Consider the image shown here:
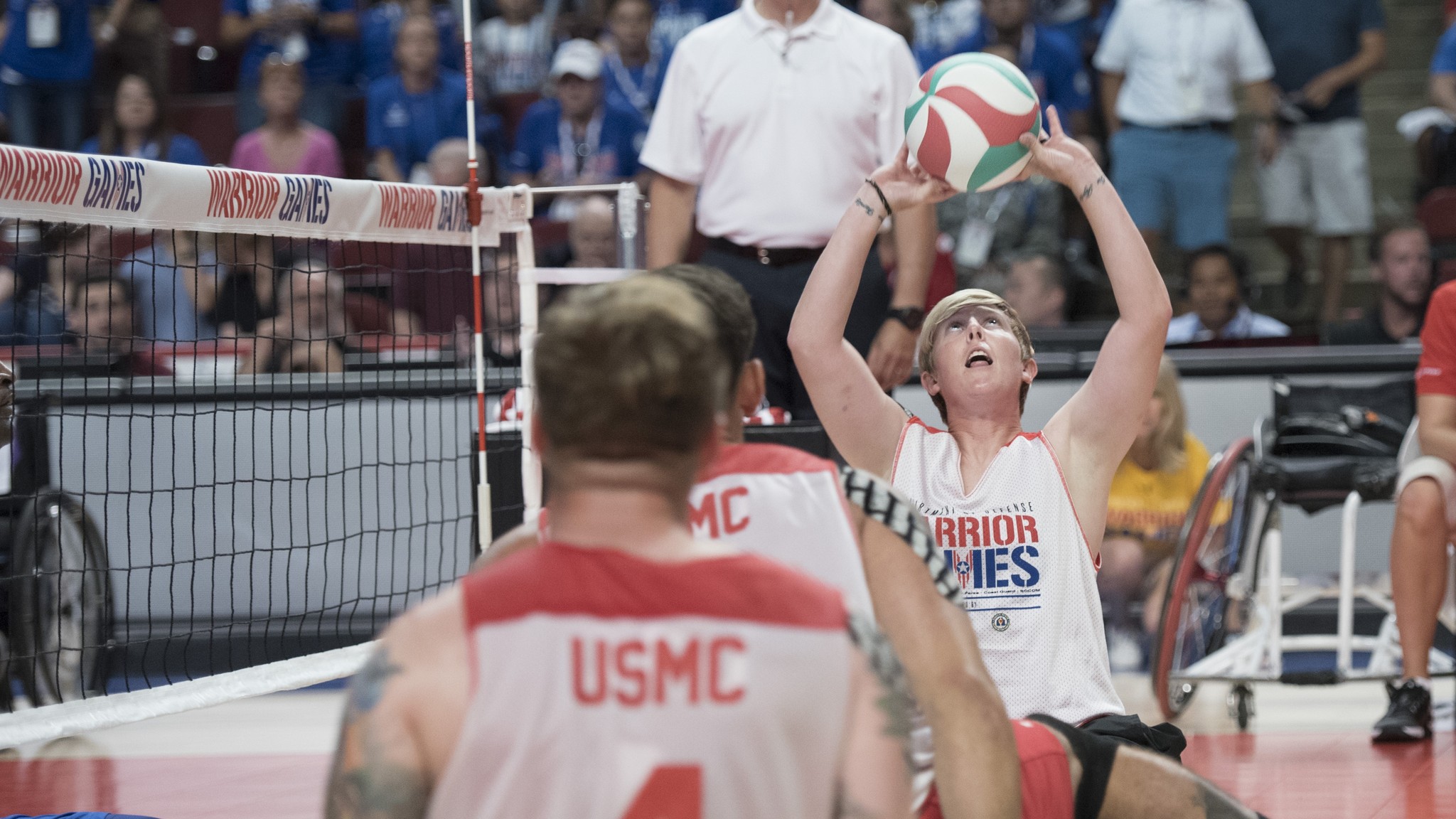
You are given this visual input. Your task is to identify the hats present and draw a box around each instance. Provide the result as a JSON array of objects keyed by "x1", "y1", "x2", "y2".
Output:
[{"x1": 547, "y1": 38, "x2": 604, "y2": 82}]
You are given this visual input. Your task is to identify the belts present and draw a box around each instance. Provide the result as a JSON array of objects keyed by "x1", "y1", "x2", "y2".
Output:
[
  {"x1": 691, "y1": 231, "x2": 879, "y2": 266},
  {"x1": 1119, "y1": 117, "x2": 1236, "y2": 135}
]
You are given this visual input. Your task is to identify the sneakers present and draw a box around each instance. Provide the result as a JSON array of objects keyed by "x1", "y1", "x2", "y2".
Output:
[{"x1": 1371, "y1": 675, "x2": 1433, "y2": 745}]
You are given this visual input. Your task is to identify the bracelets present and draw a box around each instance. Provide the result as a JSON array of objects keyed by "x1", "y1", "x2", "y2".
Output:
[{"x1": 865, "y1": 177, "x2": 892, "y2": 217}]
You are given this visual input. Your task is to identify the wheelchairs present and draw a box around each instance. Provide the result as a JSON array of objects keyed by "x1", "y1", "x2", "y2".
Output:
[
  {"x1": 1, "y1": 366, "x2": 117, "y2": 719},
  {"x1": 1149, "y1": 369, "x2": 1420, "y2": 726}
]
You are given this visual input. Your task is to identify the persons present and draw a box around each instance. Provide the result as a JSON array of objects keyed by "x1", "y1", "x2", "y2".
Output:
[
  {"x1": 226, "y1": 0, "x2": 667, "y2": 196},
  {"x1": 846, "y1": 0, "x2": 1098, "y2": 267},
  {"x1": 1169, "y1": 245, "x2": 1297, "y2": 347},
  {"x1": 1098, "y1": 350, "x2": 1234, "y2": 665},
  {"x1": 0, "y1": 0, "x2": 140, "y2": 151},
  {"x1": 782, "y1": 101, "x2": 1173, "y2": 766},
  {"x1": 1416, "y1": 19, "x2": 1456, "y2": 235},
  {"x1": 324, "y1": 275, "x2": 930, "y2": 819},
  {"x1": 999, "y1": 253, "x2": 1098, "y2": 331},
  {"x1": 1370, "y1": 277, "x2": 1456, "y2": 746},
  {"x1": 55, "y1": 275, "x2": 186, "y2": 384},
  {"x1": 250, "y1": 262, "x2": 379, "y2": 380},
  {"x1": 641, "y1": 265, "x2": 1276, "y2": 819},
  {"x1": 641, "y1": 0, "x2": 936, "y2": 474},
  {"x1": 12, "y1": 207, "x2": 296, "y2": 341},
  {"x1": 83, "y1": 76, "x2": 211, "y2": 174},
  {"x1": 1322, "y1": 223, "x2": 1435, "y2": 346},
  {"x1": 1094, "y1": 0, "x2": 1384, "y2": 333}
]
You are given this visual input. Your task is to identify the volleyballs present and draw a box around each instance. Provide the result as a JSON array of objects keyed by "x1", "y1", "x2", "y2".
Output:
[{"x1": 904, "y1": 52, "x2": 1042, "y2": 194}]
